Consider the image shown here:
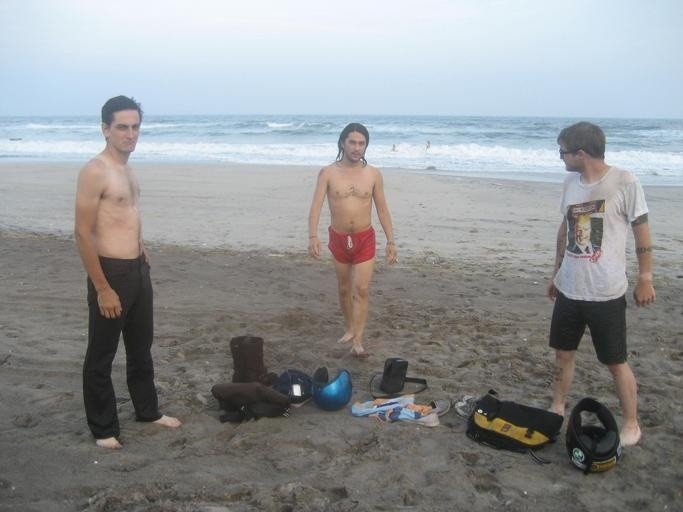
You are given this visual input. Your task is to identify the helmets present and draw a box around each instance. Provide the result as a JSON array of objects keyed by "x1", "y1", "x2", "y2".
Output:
[
  {"x1": 311, "y1": 366, "x2": 352, "y2": 412},
  {"x1": 565, "y1": 398, "x2": 622, "y2": 473}
]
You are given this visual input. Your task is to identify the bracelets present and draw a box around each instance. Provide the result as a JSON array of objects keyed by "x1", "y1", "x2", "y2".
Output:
[
  {"x1": 309, "y1": 234, "x2": 316, "y2": 240},
  {"x1": 386, "y1": 241, "x2": 395, "y2": 247}
]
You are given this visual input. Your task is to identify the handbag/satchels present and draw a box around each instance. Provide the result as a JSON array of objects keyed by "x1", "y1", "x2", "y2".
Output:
[
  {"x1": 381, "y1": 357, "x2": 408, "y2": 395},
  {"x1": 467, "y1": 395, "x2": 563, "y2": 452},
  {"x1": 210, "y1": 382, "x2": 290, "y2": 421}
]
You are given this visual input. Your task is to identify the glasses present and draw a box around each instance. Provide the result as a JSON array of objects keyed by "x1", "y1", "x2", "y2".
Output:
[{"x1": 559, "y1": 145, "x2": 583, "y2": 160}]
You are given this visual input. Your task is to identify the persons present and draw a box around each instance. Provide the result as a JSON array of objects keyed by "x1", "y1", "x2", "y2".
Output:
[
  {"x1": 307, "y1": 123, "x2": 398, "y2": 356},
  {"x1": 75, "y1": 95, "x2": 181, "y2": 450},
  {"x1": 544, "y1": 120, "x2": 656, "y2": 448}
]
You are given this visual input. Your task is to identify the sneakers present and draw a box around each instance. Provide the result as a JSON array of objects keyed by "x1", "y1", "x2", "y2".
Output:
[
  {"x1": 288, "y1": 375, "x2": 311, "y2": 408},
  {"x1": 400, "y1": 399, "x2": 451, "y2": 427},
  {"x1": 454, "y1": 388, "x2": 500, "y2": 420}
]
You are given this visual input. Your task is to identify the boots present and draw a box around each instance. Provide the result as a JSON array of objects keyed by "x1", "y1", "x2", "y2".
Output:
[{"x1": 230, "y1": 336, "x2": 278, "y2": 386}]
